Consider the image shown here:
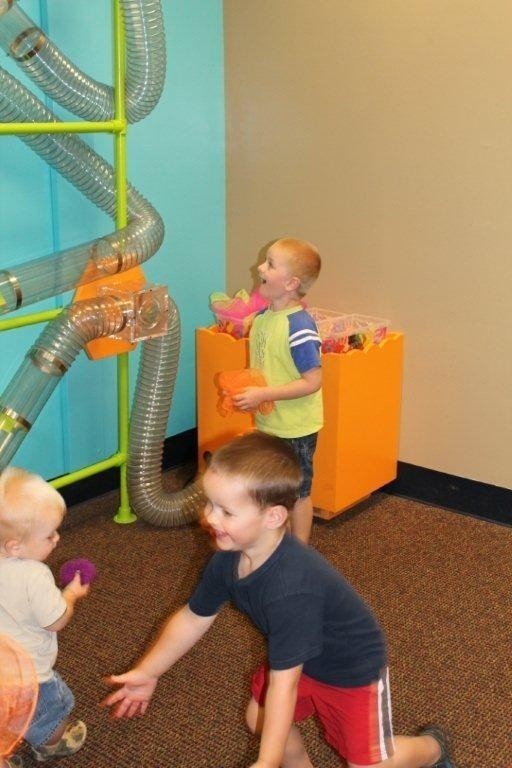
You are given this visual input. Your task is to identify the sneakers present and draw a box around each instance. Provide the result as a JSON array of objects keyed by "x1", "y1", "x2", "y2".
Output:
[
  {"x1": 6, "y1": 756, "x2": 24, "y2": 768},
  {"x1": 421, "y1": 727, "x2": 452, "y2": 768},
  {"x1": 31, "y1": 721, "x2": 87, "y2": 762}
]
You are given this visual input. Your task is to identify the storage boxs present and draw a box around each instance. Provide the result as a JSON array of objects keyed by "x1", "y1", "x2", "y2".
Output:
[{"x1": 214, "y1": 306, "x2": 391, "y2": 355}]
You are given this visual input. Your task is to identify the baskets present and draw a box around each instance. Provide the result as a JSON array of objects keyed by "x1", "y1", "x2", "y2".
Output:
[
  {"x1": 318, "y1": 314, "x2": 388, "y2": 354},
  {"x1": 307, "y1": 308, "x2": 344, "y2": 321},
  {"x1": 214, "y1": 308, "x2": 265, "y2": 337}
]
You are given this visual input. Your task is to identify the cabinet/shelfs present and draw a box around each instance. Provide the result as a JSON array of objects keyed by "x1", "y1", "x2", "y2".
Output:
[{"x1": 194, "y1": 302, "x2": 404, "y2": 528}]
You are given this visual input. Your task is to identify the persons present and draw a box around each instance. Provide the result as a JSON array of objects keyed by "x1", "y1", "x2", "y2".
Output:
[
  {"x1": 230, "y1": 236, "x2": 325, "y2": 546},
  {"x1": 106, "y1": 431, "x2": 456, "y2": 767},
  {"x1": 1, "y1": 467, "x2": 89, "y2": 761}
]
